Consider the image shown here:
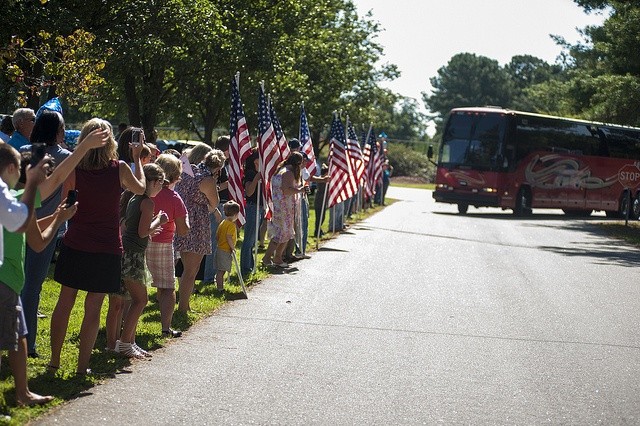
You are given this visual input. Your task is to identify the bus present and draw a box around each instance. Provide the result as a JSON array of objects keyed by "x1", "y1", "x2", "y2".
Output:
[{"x1": 427, "y1": 105, "x2": 640, "y2": 219}]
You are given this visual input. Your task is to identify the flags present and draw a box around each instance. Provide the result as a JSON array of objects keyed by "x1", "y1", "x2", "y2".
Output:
[
  {"x1": 225, "y1": 72, "x2": 269, "y2": 226},
  {"x1": 346, "y1": 117, "x2": 372, "y2": 195},
  {"x1": 267, "y1": 95, "x2": 289, "y2": 165},
  {"x1": 254, "y1": 84, "x2": 287, "y2": 223},
  {"x1": 298, "y1": 106, "x2": 317, "y2": 187},
  {"x1": 328, "y1": 113, "x2": 358, "y2": 208},
  {"x1": 361, "y1": 125, "x2": 384, "y2": 197}
]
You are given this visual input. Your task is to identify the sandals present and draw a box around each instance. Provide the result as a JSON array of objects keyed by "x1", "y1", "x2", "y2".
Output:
[
  {"x1": 132, "y1": 342, "x2": 152, "y2": 357},
  {"x1": 115, "y1": 340, "x2": 144, "y2": 361}
]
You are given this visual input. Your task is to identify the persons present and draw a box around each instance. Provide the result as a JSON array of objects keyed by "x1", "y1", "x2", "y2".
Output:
[
  {"x1": 114, "y1": 162, "x2": 171, "y2": 361},
  {"x1": 0, "y1": 154, "x2": 49, "y2": 267},
  {"x1": 382, "y1": 162, "x2": 393, "y2": 205},
  {"x1": 18, "y1": 129, "x2": 109, "y2": 208},
  {"x1": 0, "y1": 142, "x2": 79, "y2": 404},
  {"x1": 259, "y1": 150, "x2": 309, "y2": 267},
  {"x1": 103, "y1": 188, "x2": 164, "y2": 354},
  {"x1": 7, "y1": 107, "x2": 37, "y2": 149},
  {"x1": 20, "y1": 109, "x2": 71, "y2": 360},
  {"x1": 313, "y1": 162, "x2": 329, "y2": 235},
  {"x1": 114, "y1": 121, "x2": 127, "y2": 141},
  {"x1": 288, "y1": 135, "x2": 300, "y2": 150},
  {"x1": 188, "y1": 142, "x2": 210, "y2": 166},
  {"x1": 162, "y1": 147, "x2": 179, "y2": 156},
  {"x1": 213, "y1": 198, "x2": 242, "y2": 294},
  {"x1": 116, "y1": 126, "x2": 145, "y2": 178},
  {"x1": 171, "y1": 149, "x2": 227, "y2": 312},
  {"x1": 135, "y1": 144, "x2": 150, "y2": 165},
  {"x1": 327, "y1": 202, "x2": 343, "y2": 232},
  {"x1": 198, "y1": 135, "x2": 232, "y2": 285},
  {"x1": 239, "y1": 147, "x2": 266, "y2": 281},
  {"x1": 144, "y1": 152, "x2": 190, "y2": 339},
  {"x1": 43, "y1": 117, "x2": 144, "y2": 377},
  {"x1": 299, "y1": 150, "x2": 333, "y2": 258},
  {"x1": 0, "y1": 114, "x2": 14, "y2": 141}
]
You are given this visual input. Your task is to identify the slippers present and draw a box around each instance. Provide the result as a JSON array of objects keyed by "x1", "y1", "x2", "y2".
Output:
[
  {"x1": 259, "y1": 260, "x2": 274, "y2": 266},
  {"x1": 15, "y1": 394, "x2": 54, "y2": 407},
  {"x1": 273, "y1": 263, "x2": 289, "y2": 267}
]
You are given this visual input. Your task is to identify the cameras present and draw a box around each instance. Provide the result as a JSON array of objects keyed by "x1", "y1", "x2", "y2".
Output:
[{"x1": 20, "y1": 142, "x2": 57, "y2": 183}]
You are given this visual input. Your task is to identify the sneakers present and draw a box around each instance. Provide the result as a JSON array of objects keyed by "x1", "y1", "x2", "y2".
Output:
[{"x1": 162, "y1": 330, "x2": 182, "y2": 337}]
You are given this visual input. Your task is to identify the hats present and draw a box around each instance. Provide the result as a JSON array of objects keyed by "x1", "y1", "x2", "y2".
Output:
[{"x1": 288, "y1": 138, "x2": 300, "y2": 148}]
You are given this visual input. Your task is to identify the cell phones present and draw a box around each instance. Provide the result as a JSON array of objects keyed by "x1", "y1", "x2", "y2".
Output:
[
  {"x1": 86, "y1": 126, "x2": 102, "y2": 135},
  {"x1": 64, "y1": 188, "x2": 79, "y2": 208},
  {"x1": 131, "y1": 129, "x2": 141, "y2": 145}
]
